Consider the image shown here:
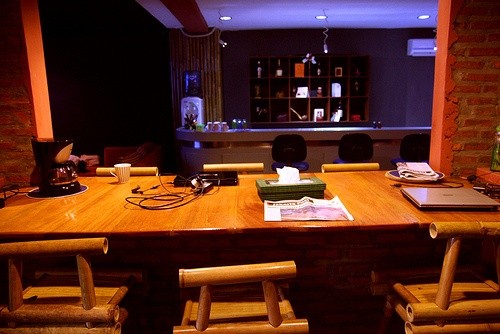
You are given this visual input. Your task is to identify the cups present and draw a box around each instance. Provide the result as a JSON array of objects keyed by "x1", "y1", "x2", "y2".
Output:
[{"x1": 109, "y1": 164, "x2": 131, "y2": 183}]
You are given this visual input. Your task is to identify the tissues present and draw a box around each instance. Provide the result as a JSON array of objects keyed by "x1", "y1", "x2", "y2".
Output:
[{"x1": 255, "y1": 165, "x2": 328, "y2": 203}]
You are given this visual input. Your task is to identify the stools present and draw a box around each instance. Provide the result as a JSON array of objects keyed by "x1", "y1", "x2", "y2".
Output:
[
  {"x1": 391, "y1": 133, "x2": 430, "y2": 165},
  {"x1": 271, "y1": 134, "x2": 309, "y2": 172},
  {"x1": 332, "y1": 133, "x2": 374, "y2": 166}
]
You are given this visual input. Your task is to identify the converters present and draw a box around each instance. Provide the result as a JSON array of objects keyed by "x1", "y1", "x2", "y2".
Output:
[{"x1": 197, "y1": 180, "x2": 213, "y2": 193}]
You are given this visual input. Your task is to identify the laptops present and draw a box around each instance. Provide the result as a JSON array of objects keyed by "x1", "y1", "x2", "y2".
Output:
[
  {"x1": 172, "y1": 169, "x2": 239, "y2": 187},
  {"x1": 401, "y1": 188, "x2": 499, "y2": 210}
]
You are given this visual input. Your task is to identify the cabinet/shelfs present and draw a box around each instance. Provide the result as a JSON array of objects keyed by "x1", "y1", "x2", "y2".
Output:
[{"x1": 248, "y1": 56, "x2": 373, "y2": 125}]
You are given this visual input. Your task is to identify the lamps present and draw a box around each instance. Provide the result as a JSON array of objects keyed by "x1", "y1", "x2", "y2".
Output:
[
  {"x1": 218, "y1": 8, "x2": 227, "y2": 47},
  {"x1": 322, "y1": 9, "x2": 329, "y2": 53}
]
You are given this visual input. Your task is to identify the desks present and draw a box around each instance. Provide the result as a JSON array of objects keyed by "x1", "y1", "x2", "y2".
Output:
[{"x1": 0, "y1": 169, "x2": 500, "y2": 295}]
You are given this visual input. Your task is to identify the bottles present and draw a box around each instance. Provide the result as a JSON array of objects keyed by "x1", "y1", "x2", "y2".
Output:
[
  {"x1": 183, "y1": 71, "x2": 201, "y2": 98},
  {"x1": 222, "y1": 122, "x2": 227, "y2": 131},
  {"x1": 276, "y1": 59, "x2": 283, "y2": 77},
  {"x1": 317, "y1": 87, "x2": 322, "y2": 97},
  {"x1": 232, "y1": 119, "x2": 247, "y2": 129},
  {"x1": 208, "y1": 122, "x2": 212, "y2": 131},
  {"x1": 317, "y1": 62, "x2": 322, "y2": 76},
  {"x1": 257, "y1": 61, "x2": 262, "y2": 78},
  {"x1": 196, "y1": 124, "x2": 204, "y2": 131},
  {"x1": 335, "y1": 60, "x2": 343, "y2": 77},
  {"x1": 214, "y1": 122, "x2": 219, "y2": 131},
  {"x1": 337, "y1": 102, "x2": 343, "y2": 121}
]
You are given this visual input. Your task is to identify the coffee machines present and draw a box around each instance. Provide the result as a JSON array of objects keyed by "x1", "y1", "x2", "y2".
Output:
[{"x1": 31, "y1": 138, "x2": 81, "y2": 197}]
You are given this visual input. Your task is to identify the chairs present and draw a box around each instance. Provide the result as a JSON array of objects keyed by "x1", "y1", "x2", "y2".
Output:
[
  {"x1": 173, "y1": 260, "x2": 309, "y2": 334},
  {"x1": 0, "y1": 238, "x2": 135, "y2": 334},
  {"x1": 370, "y1": 221, "x2": 500, "y2": 334}
]
